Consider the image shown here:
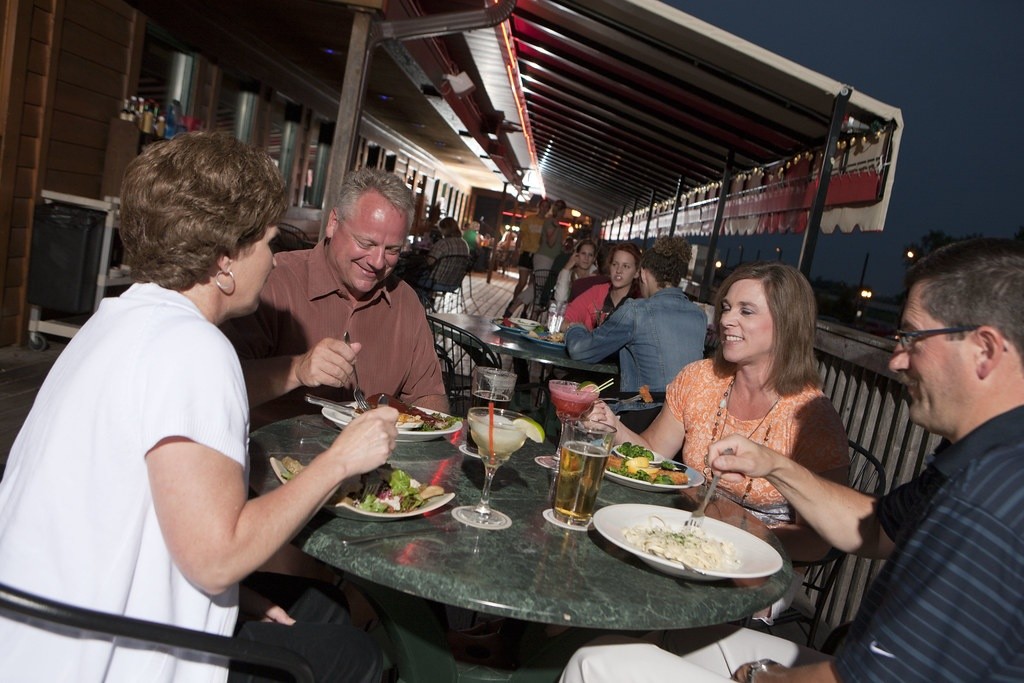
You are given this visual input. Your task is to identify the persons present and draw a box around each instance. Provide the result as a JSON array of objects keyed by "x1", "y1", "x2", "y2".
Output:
[
  {"x1": 463, "y1": 221, "x2": 481, "y2": 258},
  {"x1": 554, "y1": 239, "x2": 612, "y2": 302},
  {"x1": 561, "y1": 242, "x2": 644, "y2": 333},
  {"x1": 512, "y1": 200, "x2": 551, "y2": 320},
  {"x1": 504, "y1": 200, "x2": 567, "y2": 321},
  {"x1": 414, "y1": 217, "x2": 469, "y2": 308},
  {"x1": 565, "y1": 235, "x2": 708, "y2": 433},
  {"x1": 219, "y1": 168, "x2": 451, "y2": 578},
  {"x1": 586, "y1": 259, "x2": 848, "y2": 626},
  {"x1": 563, "y1": 236, "x2": 1024, "y2": 683},
  {"x1": 0, "y1": 130, "x2": 400, "y2": 683}
]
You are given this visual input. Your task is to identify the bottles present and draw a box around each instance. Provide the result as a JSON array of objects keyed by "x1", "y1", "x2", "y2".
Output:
[{"x1": 120, "y1": 95, "x2": 185, "y2": 153}]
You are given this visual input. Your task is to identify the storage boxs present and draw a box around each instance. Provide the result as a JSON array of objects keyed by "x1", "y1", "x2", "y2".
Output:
[{"x1": 686, "y1": 244, "x2": 721, "y2": 286}]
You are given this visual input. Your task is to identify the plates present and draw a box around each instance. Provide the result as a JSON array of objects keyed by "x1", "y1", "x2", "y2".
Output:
[
  {"x1": 321, "y1": 399, "x2": 464, "y2": 442},
  {"x1": 611, "y1": 444, "x2": 665, "y2": 465},
  {"x1": 520, "y1": 330, "x2": 567, "y2": 351},
  {"x1": 270, "y1": 455, "x2": 453, "y2": 523},
  {"x1": 592, "y1": 502, "x2": 784, "y2": 582},
  {"x1": 604, "y1": 453, "x2": 705, "y2": 492},
  {"x1": 492, "y1": 317, "x2": 523, "y2": 335}
]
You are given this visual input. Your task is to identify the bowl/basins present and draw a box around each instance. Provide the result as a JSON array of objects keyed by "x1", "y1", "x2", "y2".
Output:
[{"x1": 506, "y1": 317, "x2": 541, "y2": 331}]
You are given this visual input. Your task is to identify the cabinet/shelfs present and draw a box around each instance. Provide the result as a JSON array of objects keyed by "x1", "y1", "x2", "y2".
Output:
[
  {"x1": 99, "y1": 116, "x2": 170, "y2": 201},
  {"x1": 24, "y1": 189, "x2": 134, "y2": 339}
]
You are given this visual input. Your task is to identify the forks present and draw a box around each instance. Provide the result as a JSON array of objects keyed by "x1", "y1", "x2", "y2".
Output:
[
  {"x1": 687, "y1": 447, "x2": 733, "y2": 528},
  {"x1": 357, "y1": 393, "x2": 389, "y2": 504},
  {"x1": 342, "y1": 332, "x2": 372, "y2": 412}
]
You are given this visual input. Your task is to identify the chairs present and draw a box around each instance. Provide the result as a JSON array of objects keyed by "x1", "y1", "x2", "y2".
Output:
[{"x1": 0, "y1": 221, "x2": 887, "y2": 683}]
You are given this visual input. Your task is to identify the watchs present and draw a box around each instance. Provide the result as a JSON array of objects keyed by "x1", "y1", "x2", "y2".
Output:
[{"x1": 746, "y1": 659, "x2": 777, "y2": 683}]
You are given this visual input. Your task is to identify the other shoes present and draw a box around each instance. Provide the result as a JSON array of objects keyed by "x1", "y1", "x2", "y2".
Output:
[
  {"x1": 514, "y1": 380, "x2": 532, "y2": 393},
  {"x1": 521, "y1": 310, "x2": 527, "y2": 320}
]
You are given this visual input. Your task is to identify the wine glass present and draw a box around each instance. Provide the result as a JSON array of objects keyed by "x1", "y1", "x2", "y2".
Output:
[
  {"x1": 544, "y1": 379, "x2": 600, "y2": 472},
  {"x1": 455, "y1": 408, "x2": 525, "y2": 526}
]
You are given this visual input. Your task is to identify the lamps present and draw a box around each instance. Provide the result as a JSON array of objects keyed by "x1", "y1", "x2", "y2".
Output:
[
  {"x1": 421, "y1": 82, "x2": 443, "y2": 100},
  {"x1": 483, "y1": 110, "x2": 505, "y2": 142}
]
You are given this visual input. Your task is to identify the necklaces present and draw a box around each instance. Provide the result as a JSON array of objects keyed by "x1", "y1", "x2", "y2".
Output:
[{"x1": 704, "y1": 382, "x2": 778, "y2": 501}]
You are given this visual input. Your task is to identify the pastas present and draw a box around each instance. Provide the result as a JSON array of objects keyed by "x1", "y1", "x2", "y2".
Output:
[{"x1": 620, "y1": 515, "x2": 742, "y2": 570}]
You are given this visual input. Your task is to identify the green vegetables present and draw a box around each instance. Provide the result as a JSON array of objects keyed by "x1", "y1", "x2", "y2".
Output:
[
  {"x1": 422, "y1": 412, "x2": 464, "y2": 432},
  {"x1": 281, "y1": 463, "x2": 424, "y2": 514},
  {"x1": 629, "y1": 470, "x2": 674, "y2": 485},
  {"x1": 617, "y1": 442, "x2": 654, "y2": 461},
  {"x1": 534, "y1": 326, "x2": 545, "y2": 333},
  {"x1": 607, "y1": 457, "x2": 629, "y2": 477},
  {"x1": 660, "y1": 461, "x2": 674, "y2": 469}
]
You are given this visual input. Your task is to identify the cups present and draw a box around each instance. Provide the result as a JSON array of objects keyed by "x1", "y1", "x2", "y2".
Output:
[
  {"x1": 466, "y1": 366, "x2": 518, "y2": 454},
  {"x1": 554, "y1": 417, "x2": 618, "y2": 528}
]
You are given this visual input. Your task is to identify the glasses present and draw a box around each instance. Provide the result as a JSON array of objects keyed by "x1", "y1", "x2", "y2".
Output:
[{"x1": 894, "y1": 325, "x2": 1008, "y2": 352}]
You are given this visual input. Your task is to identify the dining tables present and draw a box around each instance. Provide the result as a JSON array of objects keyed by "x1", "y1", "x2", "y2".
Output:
[
  {"x1": 248, "y1": 401, "x2": 794, "y2": 669},
  {"x1": 426, "y1": 314, "x2": 621, "y2": 393}
]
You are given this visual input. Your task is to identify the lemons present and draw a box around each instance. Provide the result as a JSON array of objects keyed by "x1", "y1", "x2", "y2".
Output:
[
  {"x1": 577, "y1": 381, "x2": 599, "y2": 392},
  {"x1": 625, "y1": 456, "x2": 661, "y2": 475},
  {"x1": 512, "y1": 417, "x2": 545, "y2": 443}
]
